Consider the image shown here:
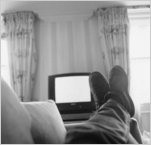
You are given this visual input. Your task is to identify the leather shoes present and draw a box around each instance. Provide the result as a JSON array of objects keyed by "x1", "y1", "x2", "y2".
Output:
[
  {"x1": 104, "y1": 66, "x2": 135, "y2": 117},
  {"x1": 89, "y1": 72, "x2": 109, "y2": 111}
]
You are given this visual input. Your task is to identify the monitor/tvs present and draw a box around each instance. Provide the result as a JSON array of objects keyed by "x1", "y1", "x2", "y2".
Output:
[{"x1": 48, "y1": 73, "x2": 96, "y2": 121}]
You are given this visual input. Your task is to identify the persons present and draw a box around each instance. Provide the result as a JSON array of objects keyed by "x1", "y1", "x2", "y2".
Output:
[{"x1": 65, "y1": 65, "x2": 138, "y2": 144}]
